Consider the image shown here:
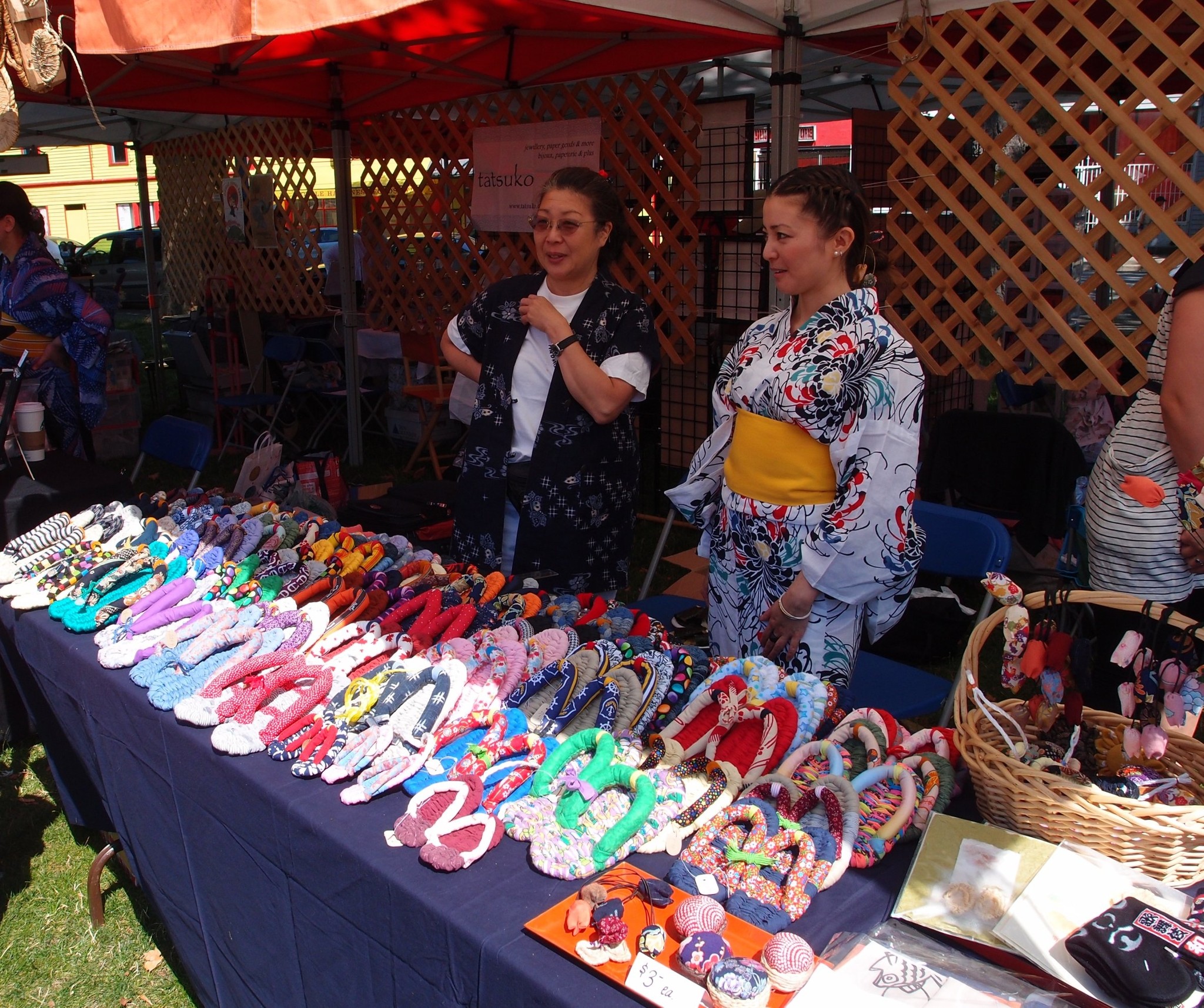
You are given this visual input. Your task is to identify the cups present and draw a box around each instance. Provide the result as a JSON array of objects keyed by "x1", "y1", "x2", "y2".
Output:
[{"x1": 15, "y1": 401, "x2": 46, "y2": 463}]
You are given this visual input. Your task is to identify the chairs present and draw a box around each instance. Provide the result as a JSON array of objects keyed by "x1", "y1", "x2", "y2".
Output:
[
  {"x1": 625, "y1": 501, "x2": 710, "y2": 634},
  {"x1": 840, "y1": 502, "x2": 1015, "y2": 740},
  {"x1": 128, "y1": 323, "x2": 474, "y2": 501}
]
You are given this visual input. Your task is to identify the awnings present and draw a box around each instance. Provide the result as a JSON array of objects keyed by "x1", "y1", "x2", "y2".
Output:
[{"x1": 65, "y1": 0, "x2": 783, "y2": 123}]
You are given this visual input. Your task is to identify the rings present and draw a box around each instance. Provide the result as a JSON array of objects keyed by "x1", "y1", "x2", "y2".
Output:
[{"x1": 1195, "y1": 557, "x2": 1202, "y2": 567}]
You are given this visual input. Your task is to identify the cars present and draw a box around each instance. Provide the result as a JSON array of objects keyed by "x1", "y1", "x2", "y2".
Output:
[
  {"x1": 386, "y1": 232, "x2": 489, "y2": 281},
  {"x1": 57, "y1": 225, "x2": 161, "y2": 304},
  {"x1": 279, "y1": 227, "x2": 356, "y2": 291}
]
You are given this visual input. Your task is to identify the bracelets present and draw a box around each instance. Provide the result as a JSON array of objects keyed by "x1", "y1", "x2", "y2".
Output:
[{"x1": 778, "y1": 589, "x2": 813, "y2": 621}]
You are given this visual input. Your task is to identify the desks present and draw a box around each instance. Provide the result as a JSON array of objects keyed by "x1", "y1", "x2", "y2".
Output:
[{"x1": 2, "y1": 486, "x2": 1204, "y2": 1008}]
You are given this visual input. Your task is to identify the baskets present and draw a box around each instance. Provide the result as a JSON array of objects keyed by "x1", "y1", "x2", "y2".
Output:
[{"x1": 956, "y1": 586, "x2": 1204, "y2": 891}]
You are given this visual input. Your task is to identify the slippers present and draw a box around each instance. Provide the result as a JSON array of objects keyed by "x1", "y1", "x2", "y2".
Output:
[{"x1": 0, "y1": 487, "x2": 958, "y2": 932}]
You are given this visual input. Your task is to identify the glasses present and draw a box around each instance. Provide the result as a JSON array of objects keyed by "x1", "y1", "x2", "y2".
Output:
[{"x1": 526, "y1": 213, "x2": 599, "y2": 238}]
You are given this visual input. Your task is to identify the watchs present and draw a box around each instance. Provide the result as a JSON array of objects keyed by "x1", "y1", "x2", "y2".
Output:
[{"x1": 549, "y1": 333, "x2": 581, "y2": 358}]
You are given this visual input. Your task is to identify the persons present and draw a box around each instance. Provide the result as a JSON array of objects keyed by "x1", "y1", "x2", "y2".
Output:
[
  {"x1": 942, "y1": 266, "x2": 1176, "y2": 578},
  {"x1": 1083, "y1": 247, "x2": 1203, "y2": 620},
  {"x1": 441, "y1": 310, "x2": 489, "y2": 456},
  {"x1": 664, "y1": 157, "x2": 926, "y2": 720},
  {"x1": 0, "y1": 179, "x2": 115, "y2": 492},
  {"x1": 48, "y1": 183, "x2": 438, "y2": 547},
  {"x1": 437, "y1": 165, "x2": 665, "y2": 607}
]
